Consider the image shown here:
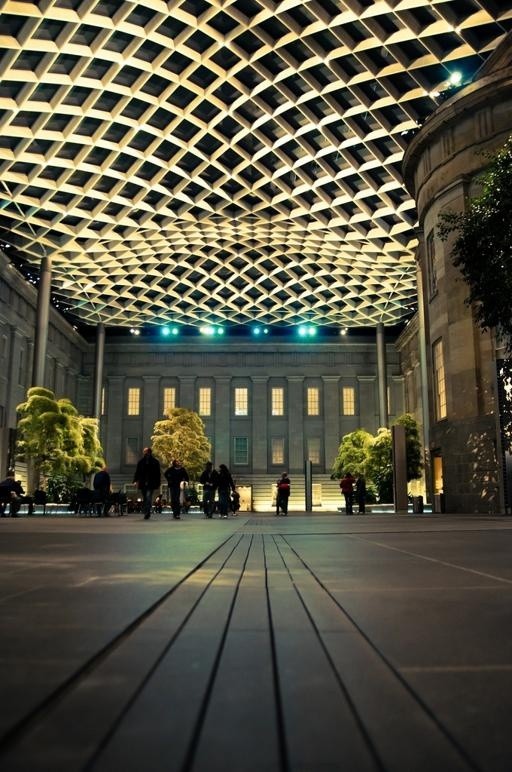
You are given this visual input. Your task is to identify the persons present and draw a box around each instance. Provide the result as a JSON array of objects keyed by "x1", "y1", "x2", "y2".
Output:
[
  {"x1": 354, "y1": 474, "x2": 368, "y2": 516},
  {"x1": 338, "y1": 471, "x2": 357, "y2": 514},
  {"x1": 156, "y1": 495, "x2": 163, "y2": 514},
  {"x1": 132, "y1": 447, "x2": 161, "y2": 519},
  {"x1": 0, "y1": 471, "x2": 25, "y2": 517},
  {"x1": 164, "y1": 459, "x2": 190, "y2": 519},
  {"x1": 275, "y1": 472, "x2": 290, "y2": 517},
  {"x1": 218, "y1": 464, "x2": 236, "y2": 519},
  {"x1": 93, "y1": 464, "x2": 113, "y2": 517},
  {"x1": 200, "y1": 461, "x2": 219, "y2": 518}
]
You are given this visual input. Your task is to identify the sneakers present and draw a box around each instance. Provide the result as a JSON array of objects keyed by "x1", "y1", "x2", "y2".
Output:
[{"x1": 144, "y1": 512, "x2": 228, "y2": 519}]
